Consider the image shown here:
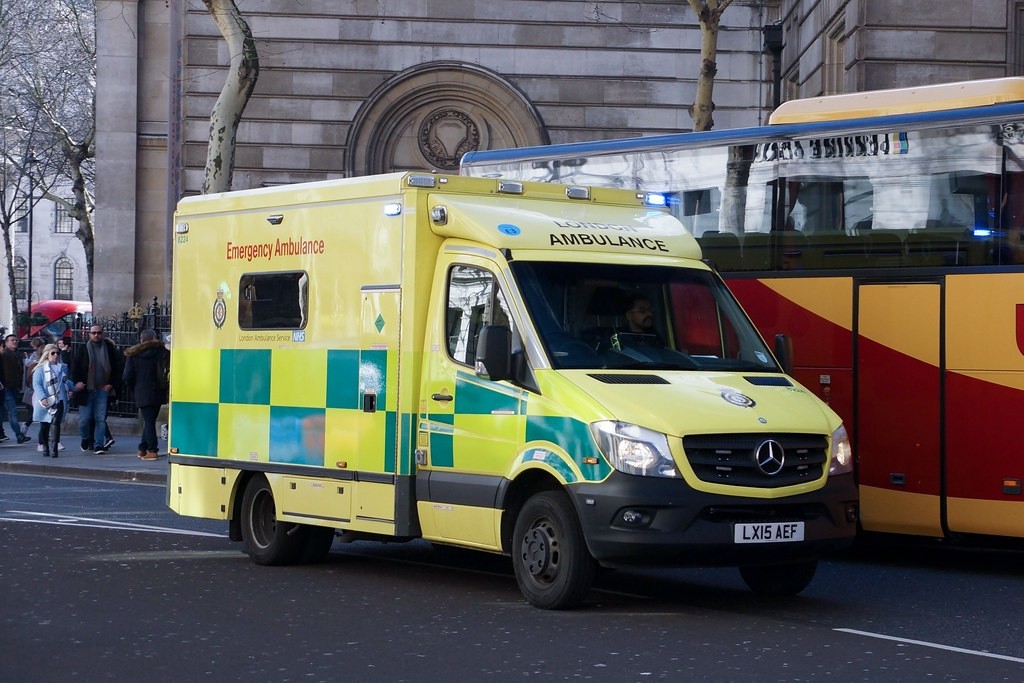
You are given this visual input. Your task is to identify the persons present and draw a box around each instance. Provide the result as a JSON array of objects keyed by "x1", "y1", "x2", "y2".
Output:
[
  {"x1": 23, "y1": 336, "x2": 44, "y2": 367},
  {"x1": 30, "y1": 343, "x2": 85, "y2": 458},
  {"x1": 57, "y1": 336, "x2": 74, "y2": 381},
  {"x1": 0, "y1": 323, "x2": 6, "y2": 341},
  {"x1": 37, "y1": 419, "x2": 66, "y2": 453},
  {"x1": 73, "y1": 323, "x2": 117, "y2": 455},
  {"x1": 121, "y1": 328, "x2": 170, "y2": 461},
  {"x1": 0, "y1": 339, "x2": 10, "y2": 442},
  {"x1": 21, "y1": 344, "x2": 47, "y2": 437},
  {"x1": 599, "y1": 292, "x2": 664, "y2": 354},
  {"x1": 2, "y1": 334, "x2": 31, "y2": 445}
]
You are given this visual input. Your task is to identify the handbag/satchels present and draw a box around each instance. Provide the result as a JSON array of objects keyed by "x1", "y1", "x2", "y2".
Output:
[{"x1": 39, "y1": 395, "x2": 55, "y2": 408}]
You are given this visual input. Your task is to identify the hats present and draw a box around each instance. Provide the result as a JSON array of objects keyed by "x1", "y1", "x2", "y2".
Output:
[{"x1": 163, "y1": 334, "x2": 171, "y2": 343}]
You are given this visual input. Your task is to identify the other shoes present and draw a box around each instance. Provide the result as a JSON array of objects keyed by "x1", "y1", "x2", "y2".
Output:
[
  {"x1": 0, "y1": 435, "x2": 9, "y2": 441},
  {"x1": 17, "y1": 436, "x2": 31, "y2": 444},
  {"x1": 21, "y1": 422, "x2": 29, "y2": 436},
  {"x1": 137, "y1": 451, "x2": 147, "y2": 458},
  {"x1": 93, "y1": 446, "x2": 105, "y2": 455},
  {"x1": 52, "y1": 452, "x2": 58, "y2": 457},
  {"x1": 161, "y1": 424, "x2": 168, "y2": 441},
  {"x1": 103, "y1": 437, "x2": 115, "y2": 451},
  {"x1": 141, "y1": 452, "x2": 158, "y2": 460},
  {"x1": 43, "y1": 450, "x2": 50, "y2": 456},
  {"x1": 58, "y1": 443, "x2": 65, "y2": 450},
  {"x1": 81, "y1": 445, "x2": 89, "y2": 452},
  {"x1": 37, "y1": 443, "x2": 44, "y2": 452}
]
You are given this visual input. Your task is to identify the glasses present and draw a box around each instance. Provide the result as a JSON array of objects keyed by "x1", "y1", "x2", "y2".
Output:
[
  {"x1": 51, "y1": 351, "x2": 60, "y2": 356},
  {"x1": 628, "y1": 307, "x2": 653, "y2": 314},
  {"x1": 90, "y1": 331, "x2": 102, "y2": 335}
]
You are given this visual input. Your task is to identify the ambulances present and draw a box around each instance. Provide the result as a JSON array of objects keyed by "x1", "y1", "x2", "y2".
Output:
[{"x1": 166, "y1": 171, "x2": 865, "y2": 610}]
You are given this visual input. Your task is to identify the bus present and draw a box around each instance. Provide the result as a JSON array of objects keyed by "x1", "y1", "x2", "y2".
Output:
[
  {"x1": 457, "y1": 75, "x2": 1024, "y2": 543},
  {"x1": 16, "y1": 300, "x2": 92, "y2": 344}
]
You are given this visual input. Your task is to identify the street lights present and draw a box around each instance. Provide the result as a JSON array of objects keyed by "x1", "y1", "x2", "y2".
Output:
[{"x1": 23, "y1": 150, "x2": 42, "y2": 338}]
